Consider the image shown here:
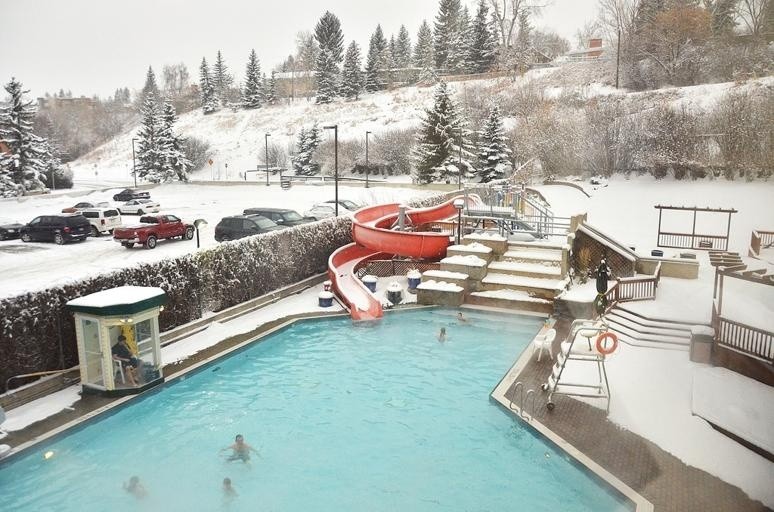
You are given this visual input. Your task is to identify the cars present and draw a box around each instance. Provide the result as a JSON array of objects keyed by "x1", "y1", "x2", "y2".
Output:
[
  {"x1": 301, "y1": 203, "x2": 351, "y2": 220},
  {"x1": 0, "y1": 222, "x2": 25, "y2": 241},
  {"x1": 115, "y1": 197, "x2": 160, "y2": 216},
  {"x1": 112, "y1": 188, "x2": 151, "y2": 202},
  {"x1": 60, "y1": 200, "x2": 110, "y2": 213},
  {"x1": 324, "y1": 199, "x2": 360, "y2": 212}
]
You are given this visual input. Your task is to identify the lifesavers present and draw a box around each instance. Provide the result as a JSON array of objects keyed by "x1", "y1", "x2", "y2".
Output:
[{"x1": 596, "y1": 333, "x2": 618, "y2": 354}]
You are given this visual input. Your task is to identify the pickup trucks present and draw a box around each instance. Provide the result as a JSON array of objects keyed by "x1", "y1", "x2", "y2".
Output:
[{"x1": 111, "y1": 213, "x2": 194, "y2": 248}]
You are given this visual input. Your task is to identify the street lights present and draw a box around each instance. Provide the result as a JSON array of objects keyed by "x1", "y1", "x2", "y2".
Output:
[
  {"x1": 130, "y1": 136, "x2": 139, "y2": 187},
  {"x1": 264, "y1": 132, "x2": 272, "y2": 185},
  {"x1": 322, "y1": 123, "x2": 339, "y2": 217},
  {"x1": 363, "y1": 130, "x2": 371, "y2": 189}
]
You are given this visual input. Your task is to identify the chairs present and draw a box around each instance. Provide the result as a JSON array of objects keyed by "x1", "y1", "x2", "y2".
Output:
[
  {"x1": 534, "y1": 328, "x2": 556, "y2": 362},
  {"x1": 581, "y1": 313, "x2": 604, "y2": 351}
]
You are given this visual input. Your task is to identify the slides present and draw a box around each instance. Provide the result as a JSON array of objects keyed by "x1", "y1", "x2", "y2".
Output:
[{"x1": 328, "y1": 194, "x2": 480, "y2": 320}]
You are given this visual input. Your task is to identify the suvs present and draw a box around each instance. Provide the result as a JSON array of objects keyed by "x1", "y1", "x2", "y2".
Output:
[
  {"x1": 242, "y1": 206, "x2": 310, "y2": 227},
  {"x1": 212, "y1": 214, "x2": 290, "y2": 242},
  {"x1": 72, "y1": 207, "x2": 122, "y2": 237}
]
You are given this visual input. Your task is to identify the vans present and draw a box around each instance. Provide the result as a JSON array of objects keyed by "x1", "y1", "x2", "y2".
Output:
[{"x1": 19, "y1": 215, "x2": 91, "y2": 244}]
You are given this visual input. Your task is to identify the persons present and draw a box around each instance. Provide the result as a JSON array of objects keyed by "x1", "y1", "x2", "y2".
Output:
[
  {"x1": 111, "y1": 335, "x2": 140, "y2": 387},
  {"x1": 218, "y1": 477, "x2": 239, "y2": 507},
  {"x1": 456, "y1": 312, "x2": 472, "y2": 327},
  {"x1": 218, "y1": 434, "x2": 264, "y2": 473},
  {"x1": 436, "y1": 327, "x2": 450, "y2": 342},
  {"x1": 123, "y1": 475, "x2": 146, "y2": 500}
]
